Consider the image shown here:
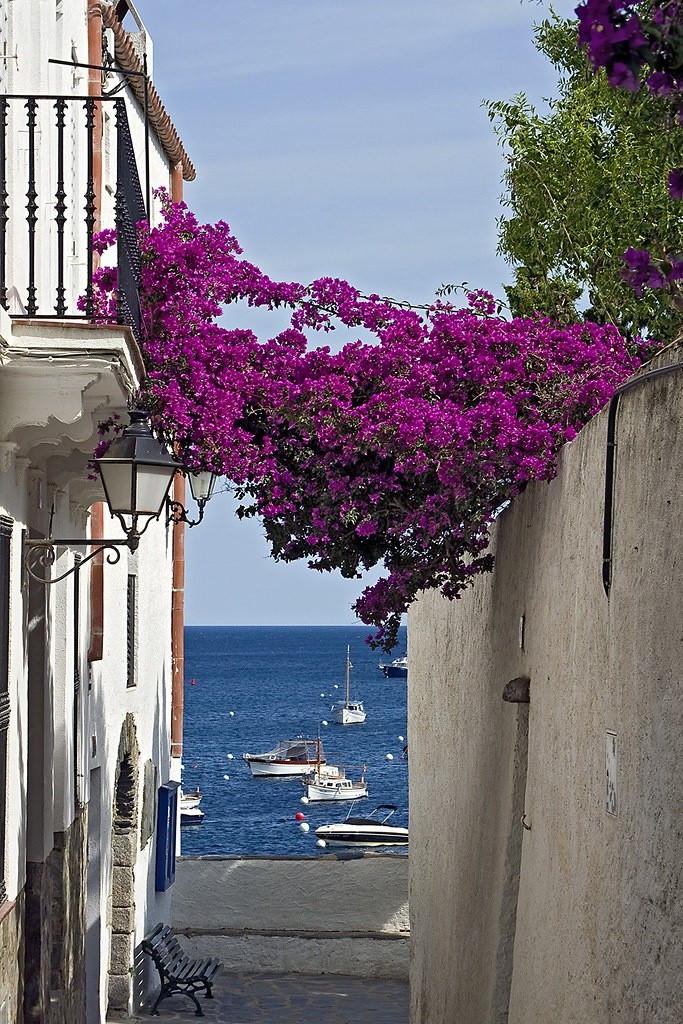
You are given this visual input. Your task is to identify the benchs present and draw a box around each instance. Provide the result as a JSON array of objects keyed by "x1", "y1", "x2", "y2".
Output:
[{"x1": 142, "y1": 922, "x2": 224, "y2": 1016}]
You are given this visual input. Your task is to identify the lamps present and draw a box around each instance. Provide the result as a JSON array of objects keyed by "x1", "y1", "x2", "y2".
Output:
[
  {"x1": 25, "y1": 389, "x2": 185, "y2": 591},
  {"x1": 166, "y1": 469, "x2": 217, "y2": 528}
]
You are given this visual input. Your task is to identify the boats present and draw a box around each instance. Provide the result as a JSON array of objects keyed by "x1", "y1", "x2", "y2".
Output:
[
  {"x1": 376, "y1": 651, "x2": 408, "y2": 678},
  {"x1": 314, "y1": 799, "x2": 410, "y2": 846},
  {"x1": 242, "y1": 738, "x2": 328, "y2": 778},
  {"x1": 300, "y1": 724, "x2": 370, "y2": 802},
  {"x1": 181, "y1": 781, "x2": 204, "y2": 826},
  {"x1": 329, "y1": 644, "x2": 367, "y2": 723}
]
